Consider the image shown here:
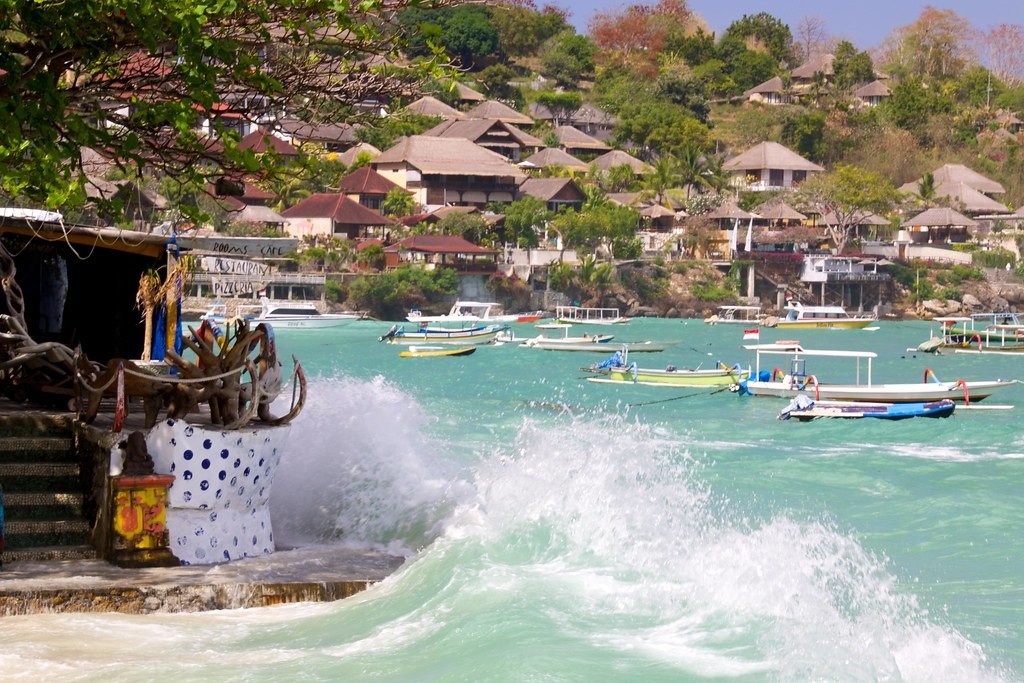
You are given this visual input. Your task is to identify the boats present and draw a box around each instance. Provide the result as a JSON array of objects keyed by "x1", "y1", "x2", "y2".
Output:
[
  {"x1": 583, "y1": 342, "x2": 752, "y2": 390},
  {"x1": 514, "y1": 312, "x2": 545, "y2": 323},
  {"x1": 518, "y1": 324, "x2": 667, "y2": 353},
  {"x1": 778, "y1": 400, "x2": 966, "y2": 426},
  {"x1": 400, "y1": 345, "x2": 479, "y2": 359},
  {"x1": 774, "y1": 291, "x2": 876, "y2": 330},
  {"x1": 405, "y1": 300, "x2": 518, "y2": 324},
  {"x1": 555, "y1": 306, "x2": 631, "y2": 325},
  {"x1": 199, "y1": 300, "x2": 233, "y2": 324},
  {"x1": 737, "y1": 326, "x2": 1019, "y2": 402},
  {"x1": 233, "y1": 287, "x2": 362, "y2": 330},
  {"x1": 907, "y1": 311, "x2": 1024, "y2": 354},
  {"x1": 705, "y1": 305, "x2": 763, "y2": 327},
  {"x1": 380, "y1": 324, "x2": 516, "y2": 346},
  {"x1": 420, "y1": 328, "x2": 526, "y2": 342}
]
